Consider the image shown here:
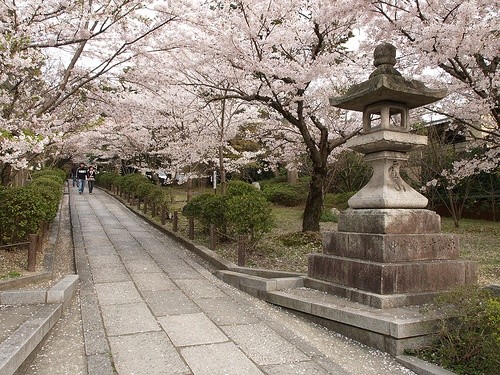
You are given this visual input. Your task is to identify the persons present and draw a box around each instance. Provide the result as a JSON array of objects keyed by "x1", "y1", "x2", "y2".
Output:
[
  {"x1": 87, "y1": 166, "x2": 96, "y2": 195},
  {"x1": 75, "y1": 162, "x2": 87, "y2": 194}
]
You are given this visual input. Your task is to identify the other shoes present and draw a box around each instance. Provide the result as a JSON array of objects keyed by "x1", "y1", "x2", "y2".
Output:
[
  {"x1": 89, "y1": 193, "x2": 93, "y2": 195},
  {"x1": 78, "y1": 191, "x2": 82, "y2": 195}
]
